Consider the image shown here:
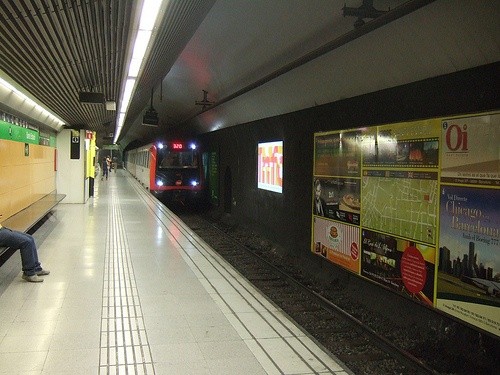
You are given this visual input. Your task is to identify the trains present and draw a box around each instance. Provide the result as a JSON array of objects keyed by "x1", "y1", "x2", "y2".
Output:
[{"x1": 123, "y1": 131, "x2": 205, "y2": 209}]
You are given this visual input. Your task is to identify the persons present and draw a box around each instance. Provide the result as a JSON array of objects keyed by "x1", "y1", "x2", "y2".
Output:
[
  {"x1": 101, "y1": 155, "x2": 112, "y2": 180},
  {"x1": 0, "y1": 215, "x2": 50, "y2": 282}
]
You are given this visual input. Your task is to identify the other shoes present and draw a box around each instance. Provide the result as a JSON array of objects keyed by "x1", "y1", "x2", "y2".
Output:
[
  {"x1": 35, "y1": 269, "x2": 49, "y2": 275},
  {"x1": 22, "y1": 273, "x2": 43, "y2": 282}
]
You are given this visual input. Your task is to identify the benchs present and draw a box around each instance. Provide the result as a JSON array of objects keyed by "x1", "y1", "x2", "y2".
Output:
[{"x1": 0, "y1": 192, "x2": 67, "y2": 257}]
